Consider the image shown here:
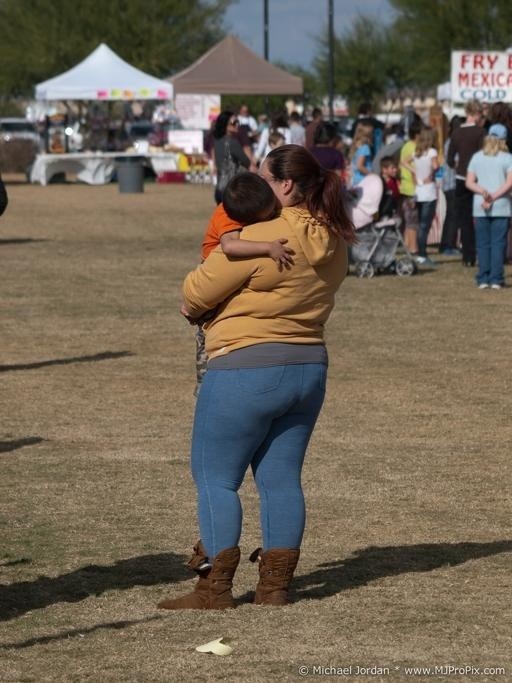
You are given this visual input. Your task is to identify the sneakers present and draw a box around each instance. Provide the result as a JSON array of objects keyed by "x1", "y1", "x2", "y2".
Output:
[
  {"x1": 479, "y1": 283, "x2": 489, "y2": 289},
  {"x1": 491, "y1": 284, "x2": 501, "y2": 289},
  {"x1": 417, "y1": 256, "x2": 436, "y2": 266}
]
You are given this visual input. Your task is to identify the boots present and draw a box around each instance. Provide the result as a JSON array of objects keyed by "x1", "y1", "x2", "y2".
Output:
[
  {"x1": 249, "y1": 546, "x2": 300, "y2": 605},
  {"x1": 157, "y1": 537, "x2": 240, "y2": 610}
]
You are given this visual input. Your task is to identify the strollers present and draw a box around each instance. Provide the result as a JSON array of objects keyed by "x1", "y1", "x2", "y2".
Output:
[{"x1": 344, "y1": 174, "x2": 417, "y2": 277}]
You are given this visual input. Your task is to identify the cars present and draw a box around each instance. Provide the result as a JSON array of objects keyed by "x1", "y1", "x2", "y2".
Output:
[{"x1": 68, "y1": 115, "x2": 153, "y2": 155}]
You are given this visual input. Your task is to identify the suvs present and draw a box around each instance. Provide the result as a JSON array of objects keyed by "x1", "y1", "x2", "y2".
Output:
[{"x1": 0, "y1": 116, "x2": 40, "y2": 146}]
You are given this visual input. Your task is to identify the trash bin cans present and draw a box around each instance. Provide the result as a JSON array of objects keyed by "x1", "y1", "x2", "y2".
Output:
[{"x1": 115, "y1": 156, "x2": 148, "y2": 193}]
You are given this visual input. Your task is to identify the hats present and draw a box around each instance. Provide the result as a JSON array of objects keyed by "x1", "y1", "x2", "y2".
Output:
[{"x1": 488, "y1": 123, "x2": 508, "y2": 139}]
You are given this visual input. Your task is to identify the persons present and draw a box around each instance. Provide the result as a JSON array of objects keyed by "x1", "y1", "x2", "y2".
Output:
[
  {"x1": 199, "y1": 103, "x2": 510, "y2": 290},
  {"x1": 190, "y1": 174, "x2": 289, "y2": 398},
  {"x1": 160, "y1": 146, "x2": 358, "y2": 606}
]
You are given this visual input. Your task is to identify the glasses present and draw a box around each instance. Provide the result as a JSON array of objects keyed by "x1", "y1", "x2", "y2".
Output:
[{"x1": 227, "y1": 119, "x2": 239, "y2": 127}]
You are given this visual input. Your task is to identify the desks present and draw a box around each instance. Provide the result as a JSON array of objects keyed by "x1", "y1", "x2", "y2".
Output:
[{"x1": 30, "y1": 152, "x2": 184, "y2": 187}]
